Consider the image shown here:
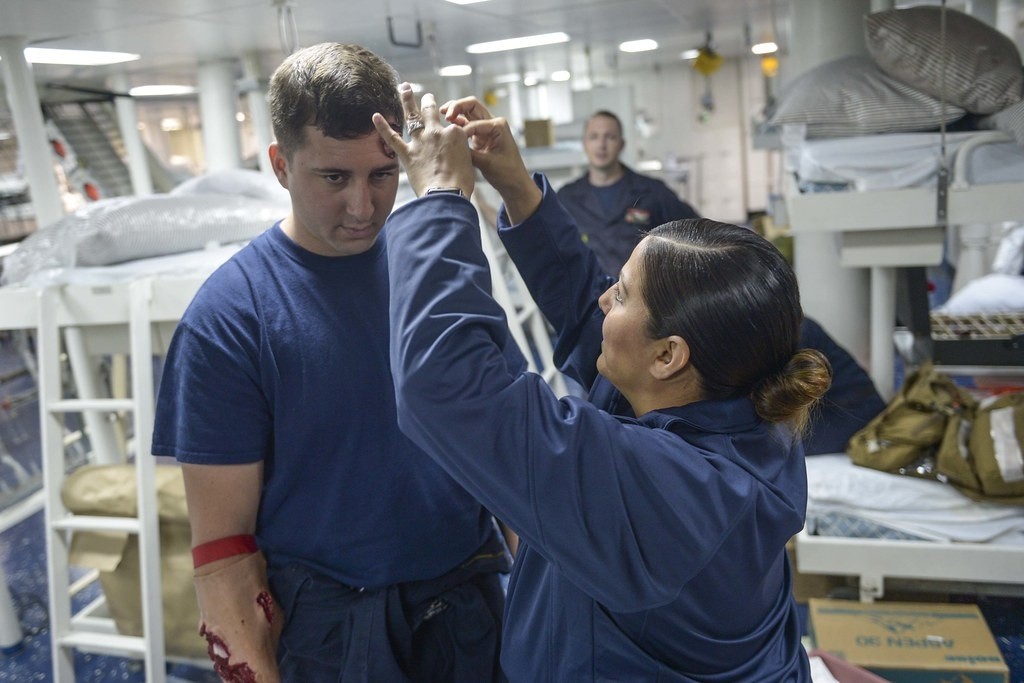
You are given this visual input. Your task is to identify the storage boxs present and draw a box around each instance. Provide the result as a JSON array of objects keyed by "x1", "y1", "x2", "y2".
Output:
[
  {"x1": 524, "y1": 119, "x2": 555, "y2": 149},
  {"x1": 61, "y1": 463, "x2": 210, "y2": 657},
  {"x1": 805, "y1": 597, "x2": 1009, "y2": 683}
]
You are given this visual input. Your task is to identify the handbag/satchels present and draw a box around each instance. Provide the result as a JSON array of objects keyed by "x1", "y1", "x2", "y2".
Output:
[{"x1": 846, "y1": 360, "x2": 1024, "y2": 505}]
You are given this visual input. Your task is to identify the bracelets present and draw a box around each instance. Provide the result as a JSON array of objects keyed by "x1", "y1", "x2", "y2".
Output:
[{"x1": 192, "y1": 534, "x2": 258, "y2": 568}]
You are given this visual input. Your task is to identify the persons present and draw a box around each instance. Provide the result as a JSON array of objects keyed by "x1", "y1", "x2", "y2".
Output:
[
  {"x1": 555, "y1": 110, "x2": 701, "y2": 284},
  {"x1": 151, "y1": 42, "x2": 521, "y2": 683},
  {"x1": 372, "y1": 82, "x2": 832, "y2": 683}
]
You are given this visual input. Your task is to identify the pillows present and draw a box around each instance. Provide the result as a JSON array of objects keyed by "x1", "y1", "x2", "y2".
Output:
[
  {"x1": 170, "y1": 168, "x2": 271, "y2": 199},
  {"x1": 863, "y1": 5, "x2": 1024, "y2": 117},
  {"x1": 945, "y1": 272, "x2": 1024, "y2": 315},
  {"x1": 0, "y1": 193, "x2": 280, "y2": 285},
  {"x1": 762, "y1": 57, "x2": 967, "y2": 140}
]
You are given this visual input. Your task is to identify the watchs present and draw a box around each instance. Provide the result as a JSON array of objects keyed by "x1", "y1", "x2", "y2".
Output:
[{"x1": 425, "y1": 187, "x2": 470, "y2": 201}]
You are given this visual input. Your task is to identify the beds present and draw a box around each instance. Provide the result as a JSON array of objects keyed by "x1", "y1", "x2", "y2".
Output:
[
  {"x1": 0, "y1": 194, "x2": 570, "y2": 683},
  {"x1": 794, "y1": 455, "x2": 1024, "y2": 602},
  {"x1": 779, "y1": 129, "x2": 1024, "y2": 232}
]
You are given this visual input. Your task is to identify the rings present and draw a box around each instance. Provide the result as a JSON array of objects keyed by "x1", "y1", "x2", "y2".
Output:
[{"x1": 408, "y1": 121, "x2": 425, "y2": 135}]
